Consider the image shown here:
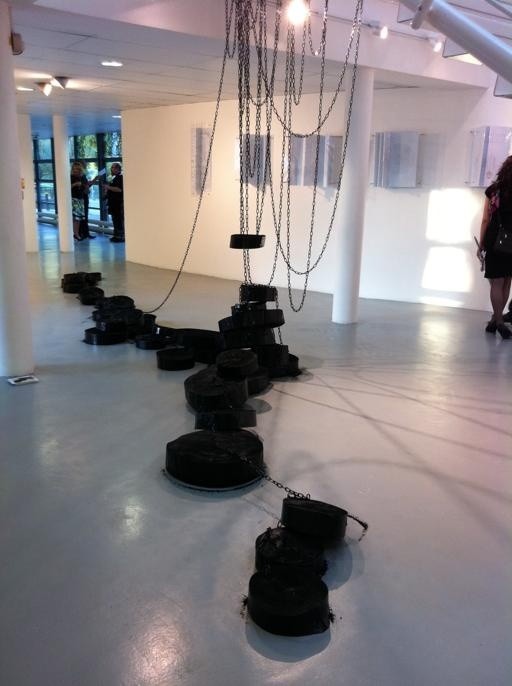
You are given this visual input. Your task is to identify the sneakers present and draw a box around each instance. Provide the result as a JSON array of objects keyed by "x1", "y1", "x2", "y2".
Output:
[
  {"x1": 486, "y1": 322, "x2": 497, "y2": 333},
  {"x1": 498, "y1": 326, "x2": 512, "y2": 339}
]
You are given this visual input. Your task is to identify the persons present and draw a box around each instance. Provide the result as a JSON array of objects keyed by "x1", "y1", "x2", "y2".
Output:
[
  {"x1": 476, "y1": 155, "x2": 512, "y2": 339},
  {"x1": 71, "y1": 158, "x2": 103, "y2": 242},
  {"x1": 100, "y1": 163, "x2": 125, "y2": 243}
]
